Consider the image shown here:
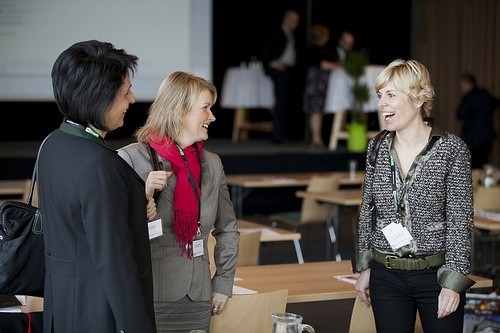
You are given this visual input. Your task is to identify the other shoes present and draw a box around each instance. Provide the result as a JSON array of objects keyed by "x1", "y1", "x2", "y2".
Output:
[{"x1": 309, "y1": 140, "x2": 326, "y2": 155}]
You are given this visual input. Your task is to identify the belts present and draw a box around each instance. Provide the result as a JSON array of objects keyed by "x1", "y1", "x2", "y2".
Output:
[{"x1": 374, "y1": 247, "x2": 445, "y2": 271}]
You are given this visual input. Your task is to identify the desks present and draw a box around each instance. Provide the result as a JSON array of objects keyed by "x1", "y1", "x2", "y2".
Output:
[
  {"x1": 473, "y1": 208, "x2": 499, "y2": 235},
  {"x1": 0, "y1": 181, "x2": 26, "y2": 196},
  {"x1": 235, "y1": 220, "x2": 304, "y2": 265},
  {"x1": 322, "y1": 64, "x2": 387, "y2": 152},
  {"x1": 226, "y1": 173, "x2": 367, "y2": 219},
  {"x1": 295, "y1": 190, "x2": 362, "y2": 262},
  {"x1": 221, "y1": 66, "x2": 275, "y2": 142},
  {"x1": 211, "y1": 260, "x2": 493, "y2": 333}
]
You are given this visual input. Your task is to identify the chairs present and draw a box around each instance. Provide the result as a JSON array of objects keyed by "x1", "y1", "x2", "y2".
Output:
[
  {"x1": 23, "y1": 181, "x2": 39, "y2": 206},
  {"x1": 207, "y1": 175, "x2": 500, "y2": 333}
]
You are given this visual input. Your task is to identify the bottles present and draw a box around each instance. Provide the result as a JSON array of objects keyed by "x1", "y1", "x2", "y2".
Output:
[{"x1": 482, "y1": 165, "x2": 496, "y2": 186}]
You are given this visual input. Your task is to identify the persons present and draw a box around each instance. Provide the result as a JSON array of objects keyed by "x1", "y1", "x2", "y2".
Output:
[
  {"x1": 116, "y1": 70, "x2": 240, "y2": 333},
  {"x1": 454, "y1": 72, "x2": 500, "y2": 171},
  {"x1": 351, "y1": 58, "x2": 477, "y2": 333},
  {"x1": 34, "y1": 39, "x2": 157, "y2": 333},
  {"x1": 262, "y1": 10, "x2": 300, "y2": 145},
  {"x1": 302, "y1": 25, "x2": 341, "y2": 148}
]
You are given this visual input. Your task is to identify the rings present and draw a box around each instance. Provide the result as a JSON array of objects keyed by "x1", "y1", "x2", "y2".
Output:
[{"x1": 213, "y1": 308, "x2": 218, "y2": 310}]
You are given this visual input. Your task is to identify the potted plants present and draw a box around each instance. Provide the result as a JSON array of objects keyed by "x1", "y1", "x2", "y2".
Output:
[{"x1": 342, "y1": 50, "x2": 370, "y2": 153}]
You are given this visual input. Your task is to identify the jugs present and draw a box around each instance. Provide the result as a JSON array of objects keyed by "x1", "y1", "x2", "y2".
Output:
[{"x1": 269, "y1": 312, "x2": 315, "y2": 333}]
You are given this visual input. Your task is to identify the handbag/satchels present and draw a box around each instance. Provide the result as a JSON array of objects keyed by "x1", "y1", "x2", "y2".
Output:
[{"x1": 0, "y1": 129, "x2": 58, "y2": 298}]
